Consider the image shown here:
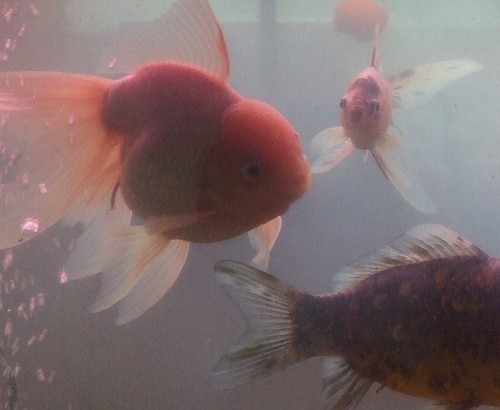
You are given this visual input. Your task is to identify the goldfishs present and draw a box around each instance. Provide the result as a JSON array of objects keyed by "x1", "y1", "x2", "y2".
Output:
[
  {"x1": 0, "y1": 0, "x2": 312, "y2": 325},
  {"x1": 312, "y1": 23, "x2": 485, "y2": 219},
  {"x1": 330, "y1": 0, "x2": 388, "y2": 36}
]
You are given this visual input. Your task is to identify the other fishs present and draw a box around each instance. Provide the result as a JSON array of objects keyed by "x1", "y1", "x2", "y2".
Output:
[{"x1": 206, "y1": 225, "x2": 500, "y2": 409}]
